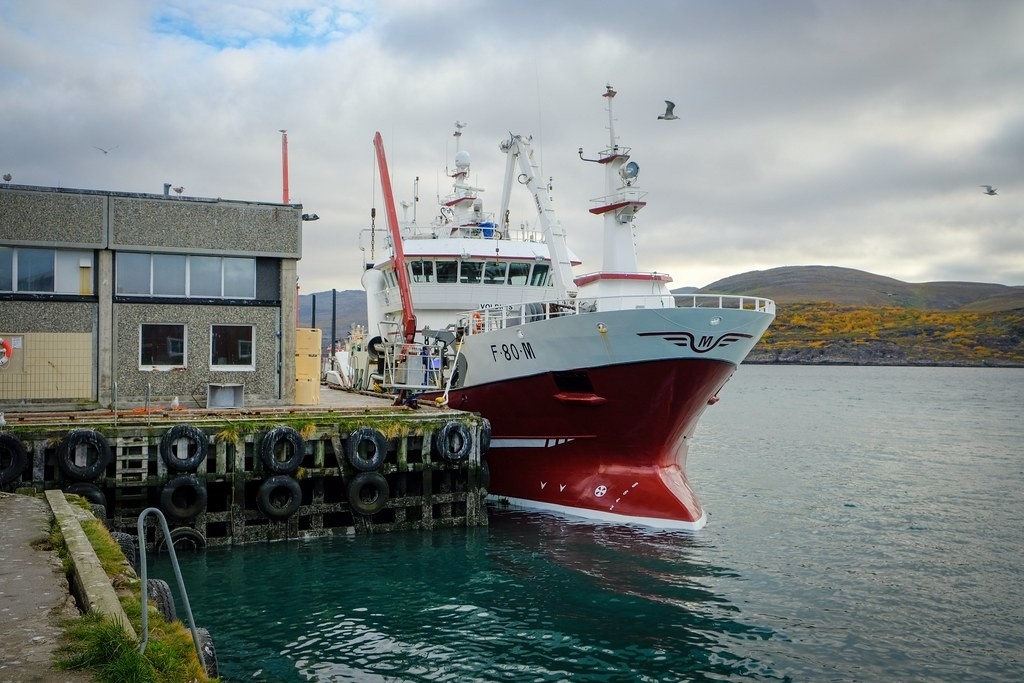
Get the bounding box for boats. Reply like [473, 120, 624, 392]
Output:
[320, 83, 776, 529]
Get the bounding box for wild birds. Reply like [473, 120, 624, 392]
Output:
[978, 185, 999, 195]
[3, 174, 12, 183]
[94, 145, 119, 153]
[173, 187, 185, 196]
[0, 412, 7, 431]
[171, 397, 179, 410]
[881, 292, 899, 296]
[658, 101, 680, 120]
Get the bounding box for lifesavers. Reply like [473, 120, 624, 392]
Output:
[259, 427, 307, 475]
[57, 428, 112, 482]
[160, 425, 209, 473]
[257, 476, 303, 518]
[0, 431, 32, 488]
[468, 311, 482, 333]
[156, 528, 208, 558]
[161, 476, 207, 522]
[346, 426, 389, 468]
[346, 472, 391, 517]
